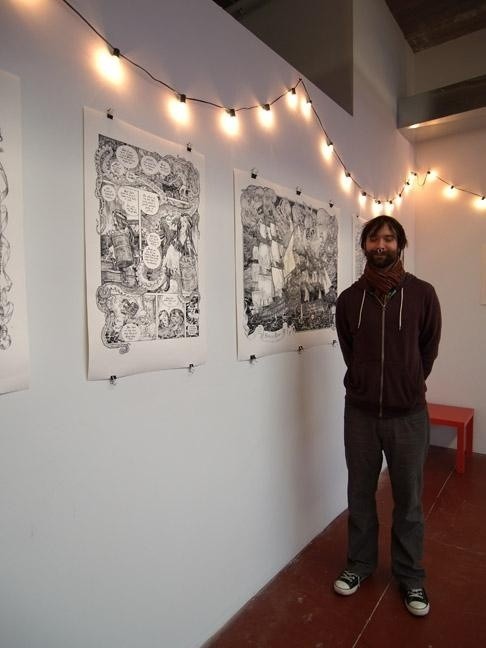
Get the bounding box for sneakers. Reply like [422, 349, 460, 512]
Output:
[334, 570, 367, 595]
[404, 585, 430, 616]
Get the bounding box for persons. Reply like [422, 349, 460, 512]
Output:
[332, 215, 443, 618]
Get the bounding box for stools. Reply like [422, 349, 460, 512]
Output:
[425, 400, 477, 475]
